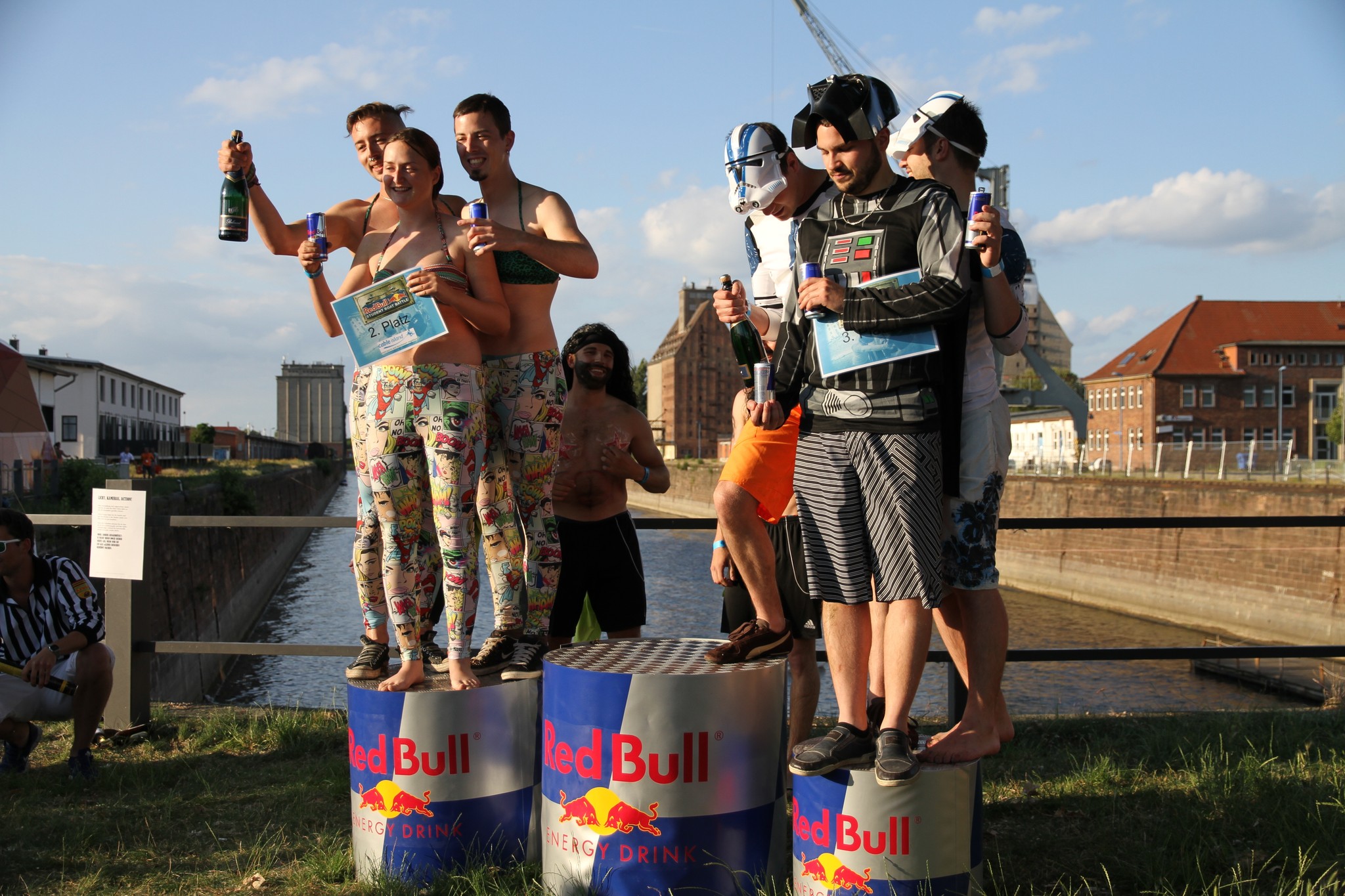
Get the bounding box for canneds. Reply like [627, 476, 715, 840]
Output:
[799, 261, 826, 319]
[754, 361, 775, 416]
[306, 212, 328, 261]
[469, 196, 489, 251]
[965, 187, 992, 250]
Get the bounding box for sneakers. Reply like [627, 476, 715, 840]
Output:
[471, 628, 524, 676]
[394, 630, 449, 673]
[501, 635, 549, 680]
[788, 722, 876, 776]
[867, 697, 919, 746]
[704, 619, 793, 665]
[874, 731, 920, 786]
[346, 634, 390, 680]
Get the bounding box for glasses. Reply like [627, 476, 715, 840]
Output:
[0, 539, 21, 553]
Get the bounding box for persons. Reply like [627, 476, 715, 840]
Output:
[219, 75, 1029, 786]
[54, 441, 158, 481]
[0, 509, 115, 784]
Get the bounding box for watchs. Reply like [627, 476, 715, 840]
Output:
[44, 643, 60, 659]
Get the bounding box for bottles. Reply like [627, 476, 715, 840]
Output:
[720, 275, 770, 387]
[218, 131, 249, 243]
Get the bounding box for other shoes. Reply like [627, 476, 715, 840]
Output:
[67, 746, 93, 781]
[0, 722, 43, 774]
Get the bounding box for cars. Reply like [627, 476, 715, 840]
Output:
[1089, 458, 1112, 475]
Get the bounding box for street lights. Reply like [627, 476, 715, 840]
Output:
[1278, 365, 1287, 475]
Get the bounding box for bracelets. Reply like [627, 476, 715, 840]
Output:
[745, 298, 751, 317]
[980, 257, 1005, 278]
[248, 176, 261, 189]
[634, 466, 649, 484]
[304, 261, 323, 279]
[244, 160, 256, 182]
[713, 539, 726, 549]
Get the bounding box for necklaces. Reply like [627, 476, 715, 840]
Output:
[841, 173, 896, 224]
[379, 191, 393, 201]
